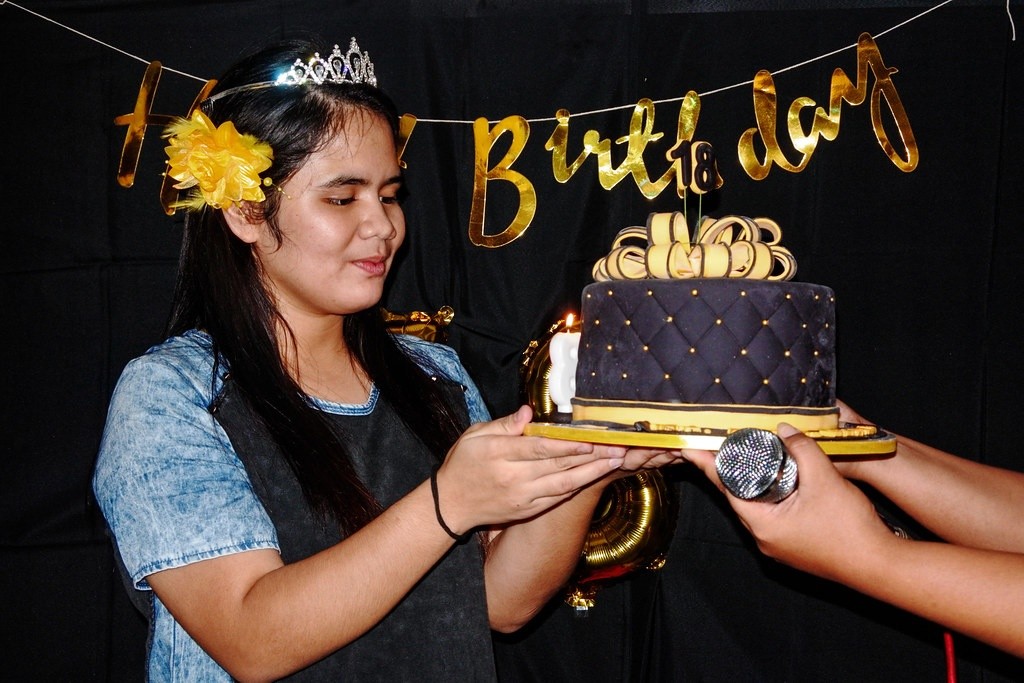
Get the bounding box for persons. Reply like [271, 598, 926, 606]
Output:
[88, 36, 687, 682]
[684, 397, 1023, 661]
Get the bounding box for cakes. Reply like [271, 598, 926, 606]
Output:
[569, 277, 840, 431]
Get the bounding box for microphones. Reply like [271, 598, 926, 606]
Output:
[716, 427, 913, 542]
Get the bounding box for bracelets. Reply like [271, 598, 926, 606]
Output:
[429, 464, 467, 541]
